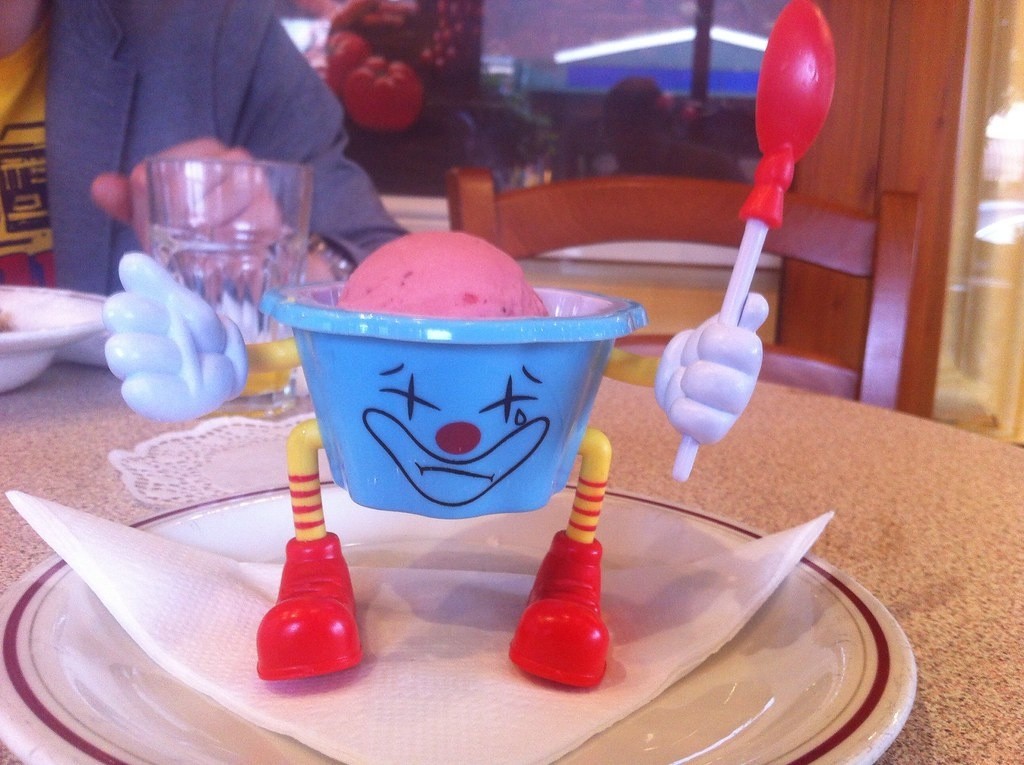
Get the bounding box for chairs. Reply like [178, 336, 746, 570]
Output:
[447, 162, 921, 409]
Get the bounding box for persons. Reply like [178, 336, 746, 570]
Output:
[0, 1, 414, 303]
[373, 70, 777, 202]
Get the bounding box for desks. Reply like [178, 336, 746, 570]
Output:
[0, 350, 1024, 765]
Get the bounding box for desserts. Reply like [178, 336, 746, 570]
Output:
[338, 231, 546, 321]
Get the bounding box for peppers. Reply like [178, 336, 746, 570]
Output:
[305, 31, 368, 96]
[345, 54, 421, 132]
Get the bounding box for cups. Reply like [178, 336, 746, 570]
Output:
[143, 151, 309, 424]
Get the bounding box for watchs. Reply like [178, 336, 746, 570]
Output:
[306, 232, 354, 282]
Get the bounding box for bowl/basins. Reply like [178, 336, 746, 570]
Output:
[262, 273, 647, 520]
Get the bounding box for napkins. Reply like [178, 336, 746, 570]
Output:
[3, 490, 838, 765]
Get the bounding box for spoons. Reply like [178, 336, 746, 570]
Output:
[674, 0, 837, 483]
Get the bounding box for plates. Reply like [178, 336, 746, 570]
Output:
[0, 284, 112, 395]
[0, 478, 923, 765]
